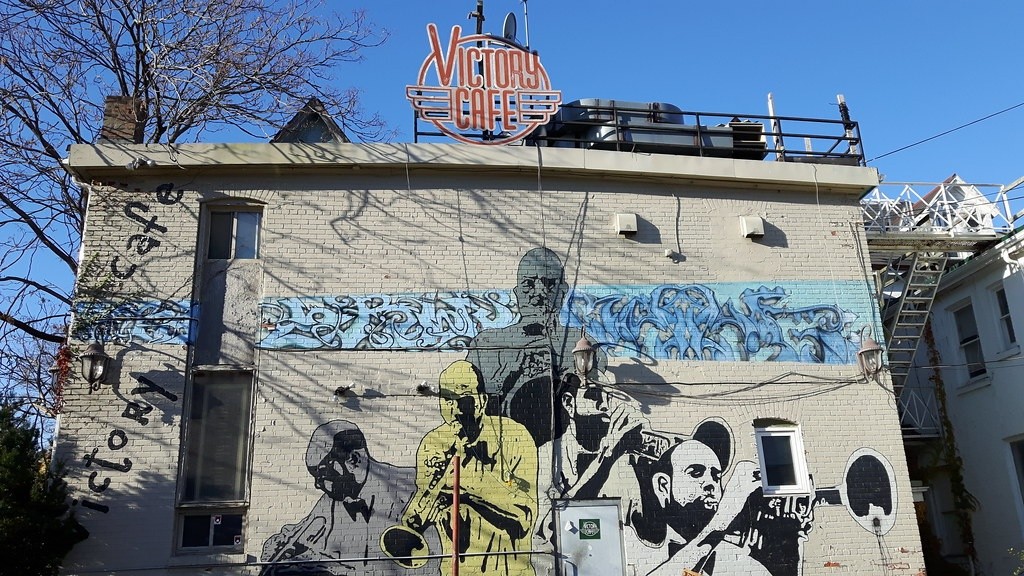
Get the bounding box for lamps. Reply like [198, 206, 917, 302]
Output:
[858, 325, 886, 382]
[572, 325, 595, 388]
[48, 356, 63, 396]
[79, 325, 112, 396]
